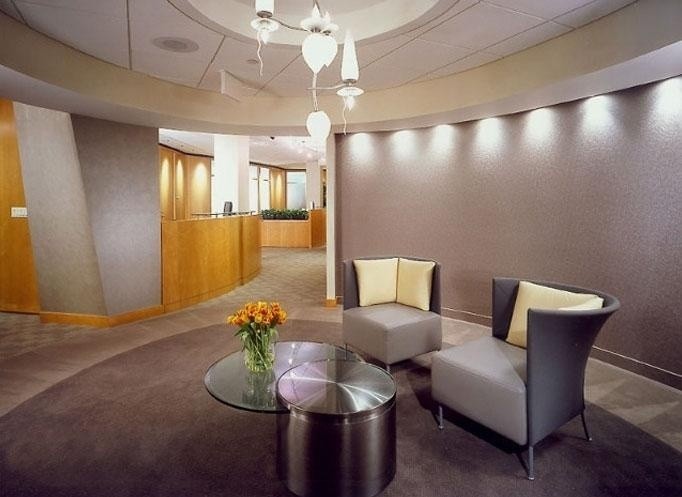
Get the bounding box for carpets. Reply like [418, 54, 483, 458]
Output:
[1, 316, 681, 497]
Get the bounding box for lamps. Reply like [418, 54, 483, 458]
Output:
[248, 0, 366, 163]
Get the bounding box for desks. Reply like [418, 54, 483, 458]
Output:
[203, 335, 399, 497]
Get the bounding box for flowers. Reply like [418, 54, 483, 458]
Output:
[224, 298, 289, 371]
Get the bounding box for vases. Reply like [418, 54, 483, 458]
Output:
[239, 329, 280, 370]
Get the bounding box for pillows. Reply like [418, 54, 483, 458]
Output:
[352, 257, 398, 306]
[559, 296, 605, 316]
[504, 279, 601, 348]
[397, 257, 438, 311]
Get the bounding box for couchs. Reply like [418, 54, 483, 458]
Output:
[341, 255, 443, 372]
[427, 275, 624, 479]
